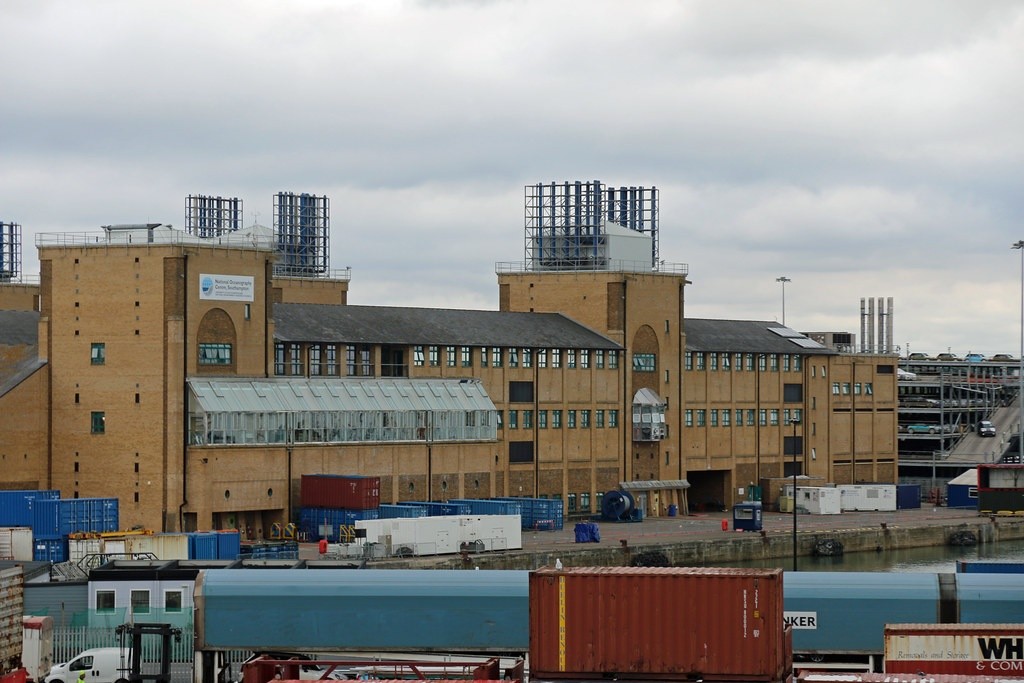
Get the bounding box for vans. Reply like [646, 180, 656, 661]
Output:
[45, 647, 142, 683]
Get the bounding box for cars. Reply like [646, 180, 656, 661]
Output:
[977, 421, 996, 437]
[937, 353, 962, 361]
[993, 354, 1021, 362]
[898, 368, 917, 381]
[898, 397, 990, 407]
[898, 423, 959, 434]
[909, 353, 934, 360]
[964, 354, 987, 362]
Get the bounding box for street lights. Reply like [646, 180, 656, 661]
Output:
[776, 277, 791, 326]
[907, 343, 909, 372]
[1011, 240, 1024, 464]
[789, 417, 799, 571]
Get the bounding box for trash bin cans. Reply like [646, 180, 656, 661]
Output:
[732, 501, 762, 532]
[668, 504, 676, 516]
[574, 523, 600, 543]
